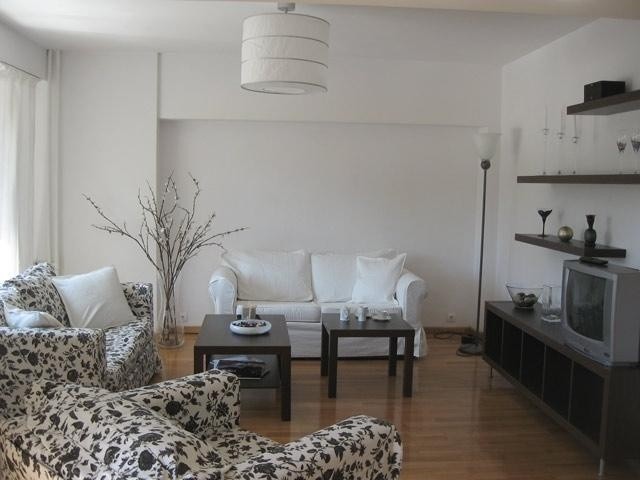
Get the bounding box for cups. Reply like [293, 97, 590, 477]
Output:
[339, 305, 352, 321]
[241, 304, 258, 320]
[375, 309, 389, 319]
[356, 306, 368, 322]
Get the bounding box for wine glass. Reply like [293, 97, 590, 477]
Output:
[615, 129, 640, 174]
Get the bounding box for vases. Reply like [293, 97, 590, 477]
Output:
[152, 267, 185, 350]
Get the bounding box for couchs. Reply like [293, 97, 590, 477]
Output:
[2, 371, 402, 480]
[0, 262, 162, 418]
[210, 248, 430, 359]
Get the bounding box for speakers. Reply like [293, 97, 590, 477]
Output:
[584, 81, 625, 103]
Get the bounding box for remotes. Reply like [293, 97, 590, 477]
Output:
[580, 256, 608, 265]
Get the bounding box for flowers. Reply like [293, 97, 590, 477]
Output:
[83, 167, 250, 276]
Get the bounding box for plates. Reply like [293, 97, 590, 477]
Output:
[374, 316, 392, 321]
[354, 313, 372, 318]
[236, 314, 260, 319]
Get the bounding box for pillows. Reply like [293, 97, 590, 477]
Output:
[48, 265, 137, 331]
[350, 253, 409, 306]
[1, 300, 61, 329]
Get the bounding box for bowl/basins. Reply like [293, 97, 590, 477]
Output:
[230, 319, 271, 335]
[504, 282, 543, 307]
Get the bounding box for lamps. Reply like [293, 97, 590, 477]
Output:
[237, 1, 331, 97]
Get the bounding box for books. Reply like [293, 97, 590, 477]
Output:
[207, 357, 271, 381]
[210, 356, 271, 376]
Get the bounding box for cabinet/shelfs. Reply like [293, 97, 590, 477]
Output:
[512, 83, 639, 261]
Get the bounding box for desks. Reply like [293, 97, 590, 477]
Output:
[484, 299, 639, 478]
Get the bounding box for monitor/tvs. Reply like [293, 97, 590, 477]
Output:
[561, 261, 640, 367]
